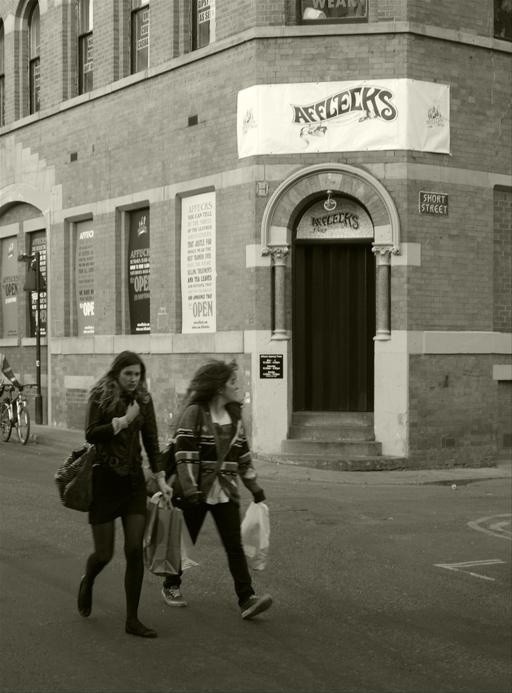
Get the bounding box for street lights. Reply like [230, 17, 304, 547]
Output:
[15, 250, 45, 423]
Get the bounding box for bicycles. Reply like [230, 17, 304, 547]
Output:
[0, 383, 41, 445]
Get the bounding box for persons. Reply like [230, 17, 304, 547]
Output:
[1, 353, 24, 416]
[161, 356, 272, 619]
[76, 349, 164, 639]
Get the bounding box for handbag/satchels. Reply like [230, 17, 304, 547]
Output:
[54, 442, 97, 514]
[237, 500, 272, 573]
[142, 492, 201, 576]
[144, 441, 203, 508]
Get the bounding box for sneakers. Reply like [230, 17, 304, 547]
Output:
[124, 619, 157, 639]
[77, 572, 96, 618]
[240, 592, 274, 620]
[161, 585, 188, 607]
